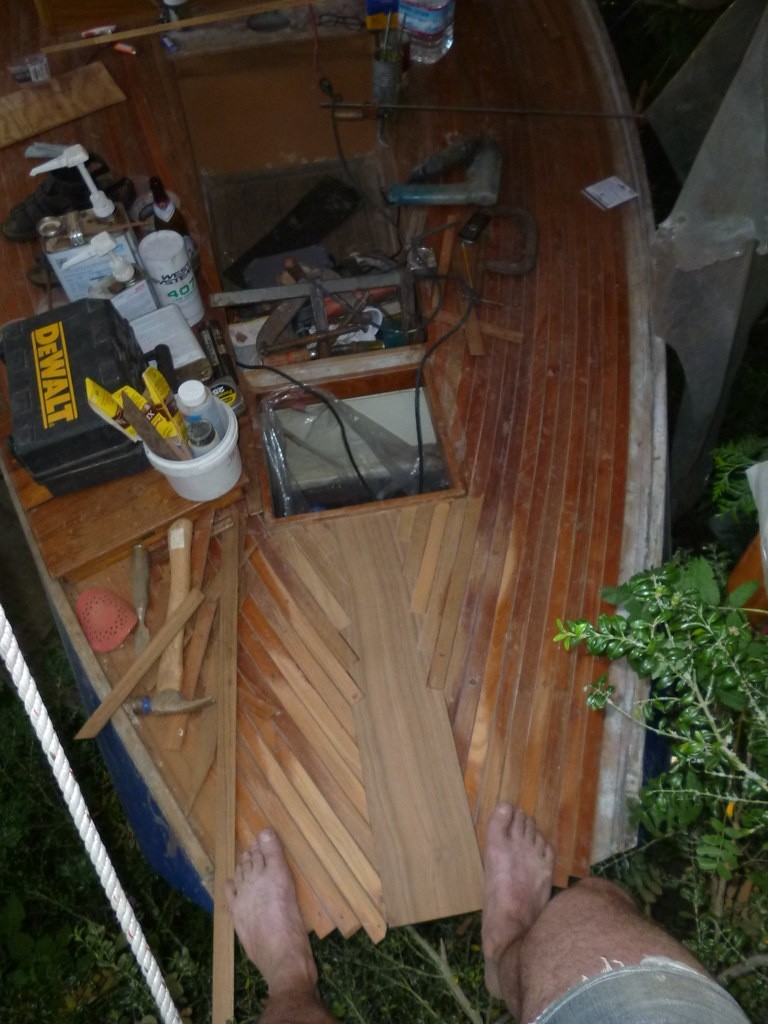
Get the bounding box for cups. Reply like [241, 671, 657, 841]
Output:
[130, 190, 180, 234]
[25, 53, 51, 85]
[6, 57, 31, 87]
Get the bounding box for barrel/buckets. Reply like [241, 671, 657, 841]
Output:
[143, 392, 242, 502]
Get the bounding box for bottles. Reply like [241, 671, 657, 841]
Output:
[398, 0, 455, 64]
[150, 175, 201, 274]
[176, 380, 226, 441]
[188, 421, 220, 457]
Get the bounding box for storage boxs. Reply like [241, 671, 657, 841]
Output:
[0, 298, 149, 497]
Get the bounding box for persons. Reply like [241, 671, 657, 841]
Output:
[226, 803, 750, 1024]
